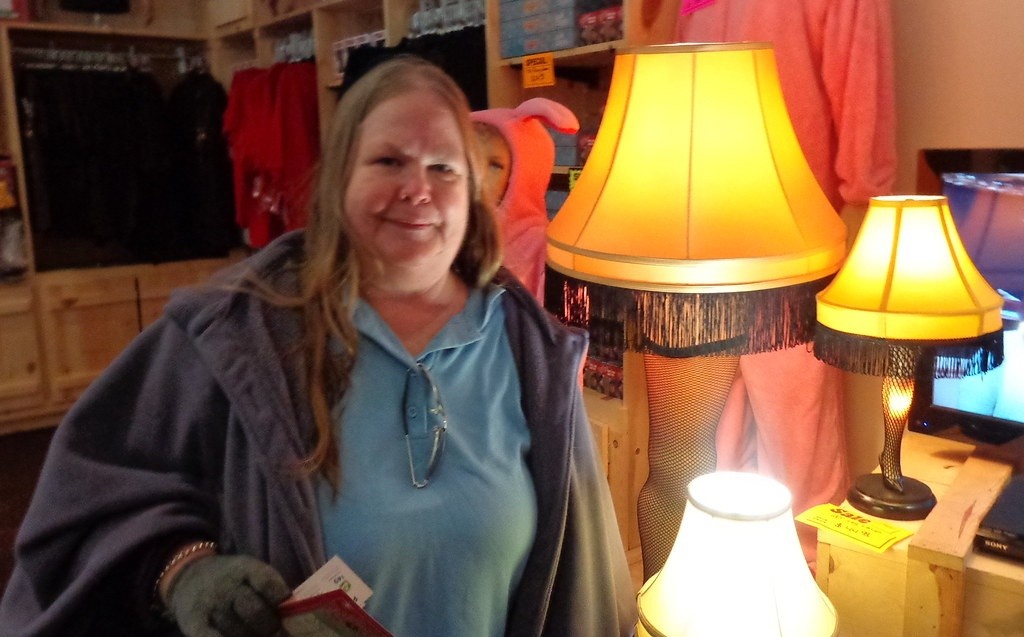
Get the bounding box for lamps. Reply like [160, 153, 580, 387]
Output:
[540, 39, 847, 598]
[809, 192, 1004, 521]
[632, 469, 841, 637]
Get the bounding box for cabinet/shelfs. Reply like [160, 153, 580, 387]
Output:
[0, 0, 679, 557]
[814, 429, 1023, 637]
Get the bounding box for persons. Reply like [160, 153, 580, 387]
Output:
[0, 59, 638, 637]
[469, 98, 581, 305]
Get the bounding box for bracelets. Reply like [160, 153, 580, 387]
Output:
[153, 538, 216, 593]
